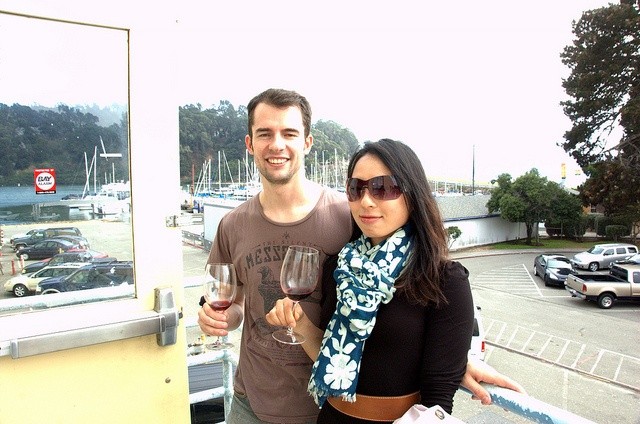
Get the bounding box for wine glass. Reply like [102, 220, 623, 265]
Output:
[271, 245, 320, 345]
[203, 263, 238, 350]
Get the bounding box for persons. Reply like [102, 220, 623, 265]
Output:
[265, 139, 474, 424]
[197, 89, 526, 424]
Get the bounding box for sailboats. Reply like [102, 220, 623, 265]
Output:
[429, 180, 484, 198]
[182, 151, 266, 212]
[34, 135, 130, 223]
[306, 149, 350, 193]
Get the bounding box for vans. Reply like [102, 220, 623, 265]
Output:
[569, 243, 638, 273]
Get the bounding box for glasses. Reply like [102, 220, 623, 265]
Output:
[345, 174, 401, 201]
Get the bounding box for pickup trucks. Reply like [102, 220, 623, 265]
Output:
[562, 264, 640, 307]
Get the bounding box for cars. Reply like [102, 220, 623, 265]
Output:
[607, 251, 640, 273]
[534, 254, 573, 289]
[5, 224, 134, 298]
